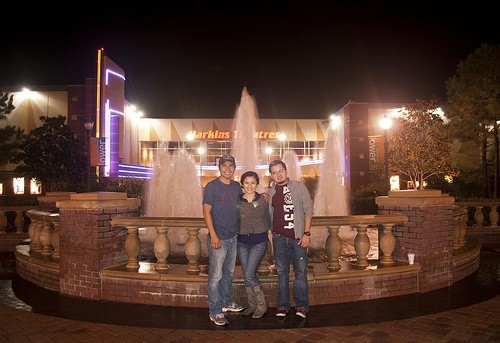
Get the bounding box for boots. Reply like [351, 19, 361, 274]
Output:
[252, 284, 267, 319]
[242, 287, 258, 316]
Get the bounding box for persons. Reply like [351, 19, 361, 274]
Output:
[237, 171, 274, 318]
[267, 160, 314, 319]
[202, 154, 270, 325]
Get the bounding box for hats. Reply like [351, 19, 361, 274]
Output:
[219, 154, 234, 164]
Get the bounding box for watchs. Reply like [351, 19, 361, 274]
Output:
[304, 231, 311, 236]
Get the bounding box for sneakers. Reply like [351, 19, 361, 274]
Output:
[210, 312, 229, 326]
[221, 302, 244, 312]
[296, 307, 306, 318]
[275, 307, 287, 317]
